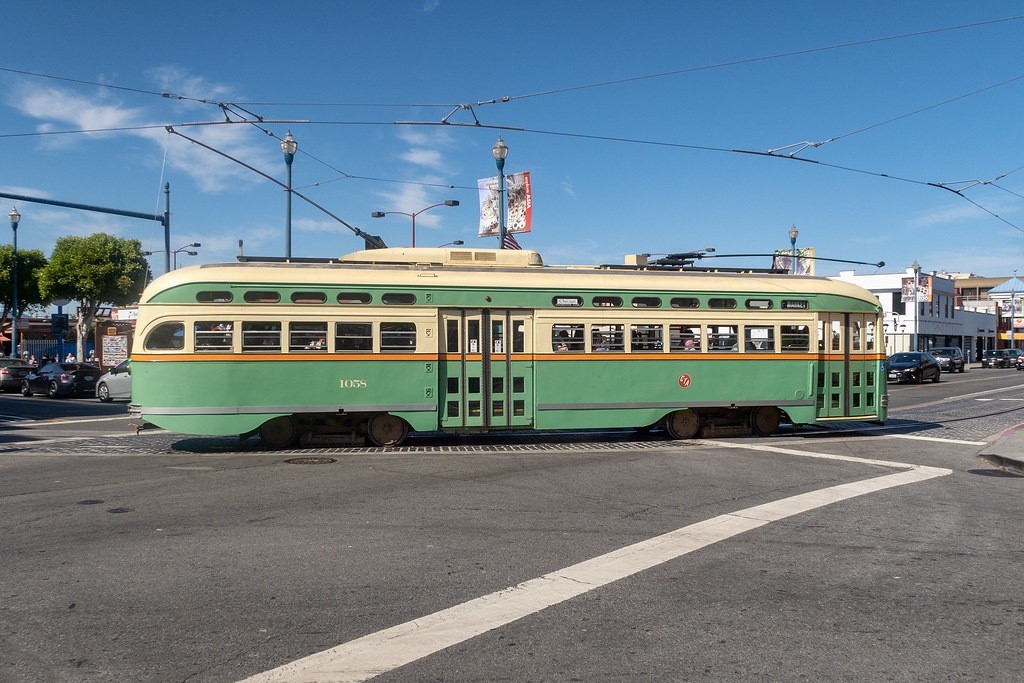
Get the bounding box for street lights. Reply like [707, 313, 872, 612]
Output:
[1011, 289, 1015, 350]
[371, 200, 460, 248]
[142, 242, 201, 271]
[8, 205, 21, 358]
[491, 132, 508, 249]
[912, 259, 919, 351]
[280, 129, 298, 258]
[789, 223, 799, 275]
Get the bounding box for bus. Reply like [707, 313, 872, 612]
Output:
[127, 125, 890, 447]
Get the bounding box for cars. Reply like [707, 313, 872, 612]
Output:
[1006, 348, 1024, 371]
[95, 359, 131, 403]
[0, 358, 37, 393]
[20, 362, 102, 400]
[982, 350, 1010, 369]
[886, 352, 941, 385]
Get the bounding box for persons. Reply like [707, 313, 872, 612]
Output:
[555, 326, 809, 351]
[28, 353, 75, 367]
[198, 323, 414, 350]
[0, 344, 5, 358]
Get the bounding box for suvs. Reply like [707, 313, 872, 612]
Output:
[928, 347, 965, 373]
